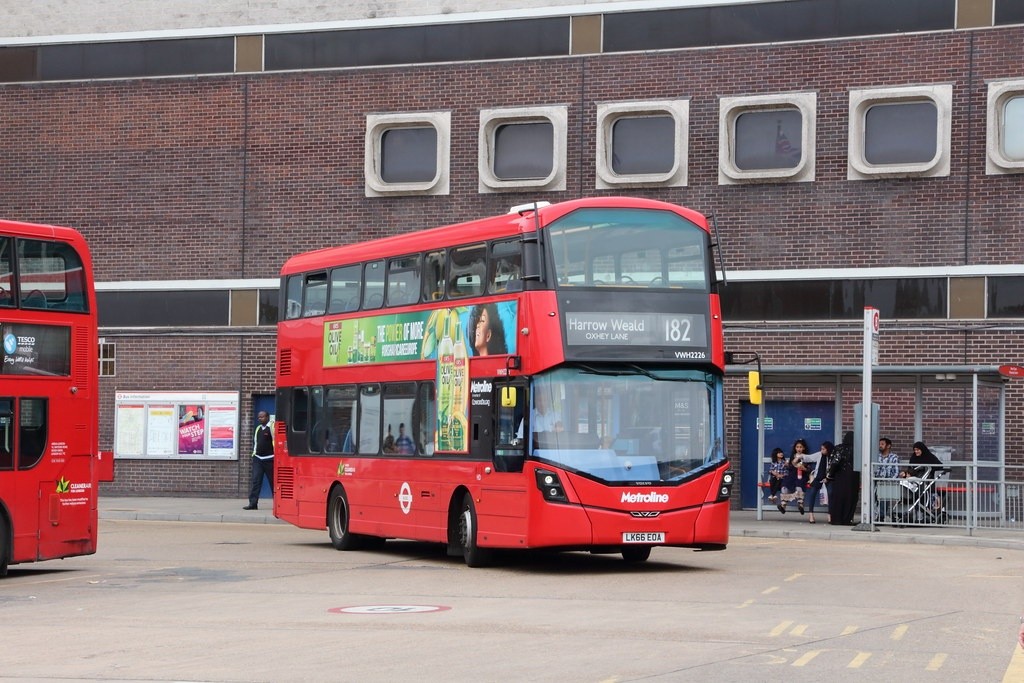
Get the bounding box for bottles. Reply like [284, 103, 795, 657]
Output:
[792, 462, 806, 470]
[449, 322, 469, 453]
[435, 317, 454, 454]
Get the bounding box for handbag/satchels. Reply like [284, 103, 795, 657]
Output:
[832, 458, 851, 491]
[819, 482, 829, 506]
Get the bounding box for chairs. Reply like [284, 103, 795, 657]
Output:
[0, 286, 87, 312]
[308, 292, 418, 314]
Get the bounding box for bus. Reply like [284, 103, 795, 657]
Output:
[0, 220, 100, 576]
[273, 197, 765, 568]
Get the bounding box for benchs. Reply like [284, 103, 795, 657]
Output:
[760, 481, 810, 491]
[934, 486, 997, 495]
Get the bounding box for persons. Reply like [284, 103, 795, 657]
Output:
[822, 431, 860, 525]
[777, 439, 812, 515]
[875, 438, 900, 528]
[768, 448, 788, 504]
[899, 441, 943, 527]
[383, 423, 414, 454]
[796, 441, 834, 524]
[242, 412, 275, 509]
[469, 302, 508, 356]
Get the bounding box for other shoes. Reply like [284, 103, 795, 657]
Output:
[242, 503, 258, 510]
[828, 515, 832, 525]
[809, 516, 815, 523]
[799, 505, 804, 515]
[777, 504, 786, 514]
[768, 495, 777, 504]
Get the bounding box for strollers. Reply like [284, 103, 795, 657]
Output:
[891, 466, 951, 526]
